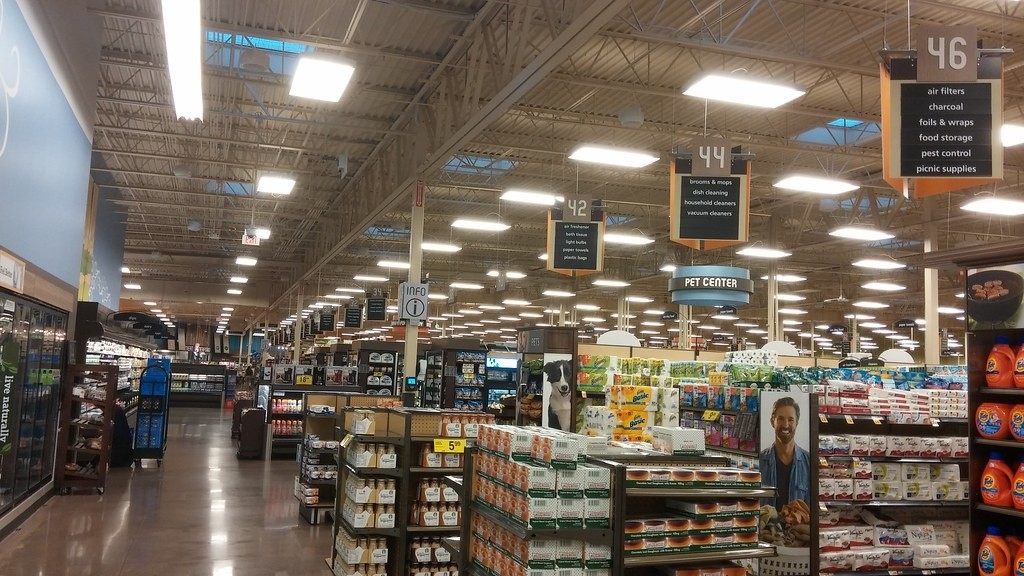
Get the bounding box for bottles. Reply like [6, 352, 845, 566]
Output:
[272, 420, 302, 435]
[412, 536, 441, 548]
[345, 497, 395, 514]
[443, 413, 495, 423]
[353, 410, 375, 421]
[338, 526, 386, 549]
[410, 561, 458, 573]
[981, 452, 1014, 508]
[411, 500, 461, 512]
[420, 443, 431, 454]
[986, 336, 1024, 389]
[1012, 455, 1024, 512]
[347, 471, 395, 490]
[978, 527, 1024, 576]
[272, 399, 302, 414]
[336, 553, 386, 574]
[419, 478, 448, 488]
[130, 396, 138, 405]
[350, 442, 395, 454]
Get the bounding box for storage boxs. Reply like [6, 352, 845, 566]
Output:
[652, 425, 705, 455]
[472, 426, 609, 576]
[135, 358, 172, 449]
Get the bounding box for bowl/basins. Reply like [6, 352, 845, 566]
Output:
[968, 270, 1024, 324]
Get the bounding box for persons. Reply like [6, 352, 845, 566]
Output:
[759, 397, 810, 513]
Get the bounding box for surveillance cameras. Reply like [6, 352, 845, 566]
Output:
[246, 229, 256, 238]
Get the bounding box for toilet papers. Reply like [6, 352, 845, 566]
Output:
[576, 354, 775, 393]
[583, 385, 680, 442]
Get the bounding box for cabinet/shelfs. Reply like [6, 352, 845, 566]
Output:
[587, 454, 778, 576]
[168, 363, 227, 409]
[299, 392, 414, 526]
[54, 365, 119, 494]
[949, 238, 1024, 576]
[77, 352, 148, 445]
[760, 391, 969, 576]
[258, 383, 361, 461]
[681, 406, 759, 457]
[325, 406, 478, 576]
[423, 339, 517, 424]
[351, 341, 430, 407]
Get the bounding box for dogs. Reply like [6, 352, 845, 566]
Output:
[544, 359, 573, 432]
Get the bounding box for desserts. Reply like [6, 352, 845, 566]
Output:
[624, 471, 760, 549]
